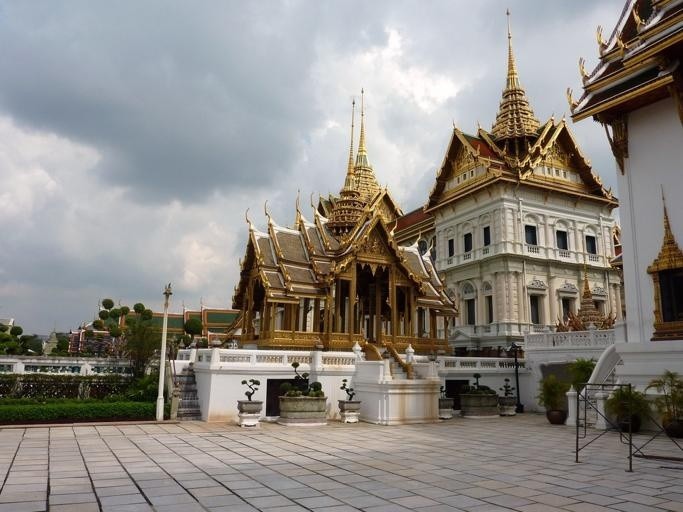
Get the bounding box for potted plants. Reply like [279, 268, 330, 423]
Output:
[459, 374, 498, 413]
[536, 375, 572, 424]
[338, 379, 362, 413]
[605, 382, 651, 433]
[237, 379, 264, 413]
[646, 369, 683, 438]
[279, 362, 329, 418]
[499, 378, 518, 406]
[439, 386, 455, 408]
